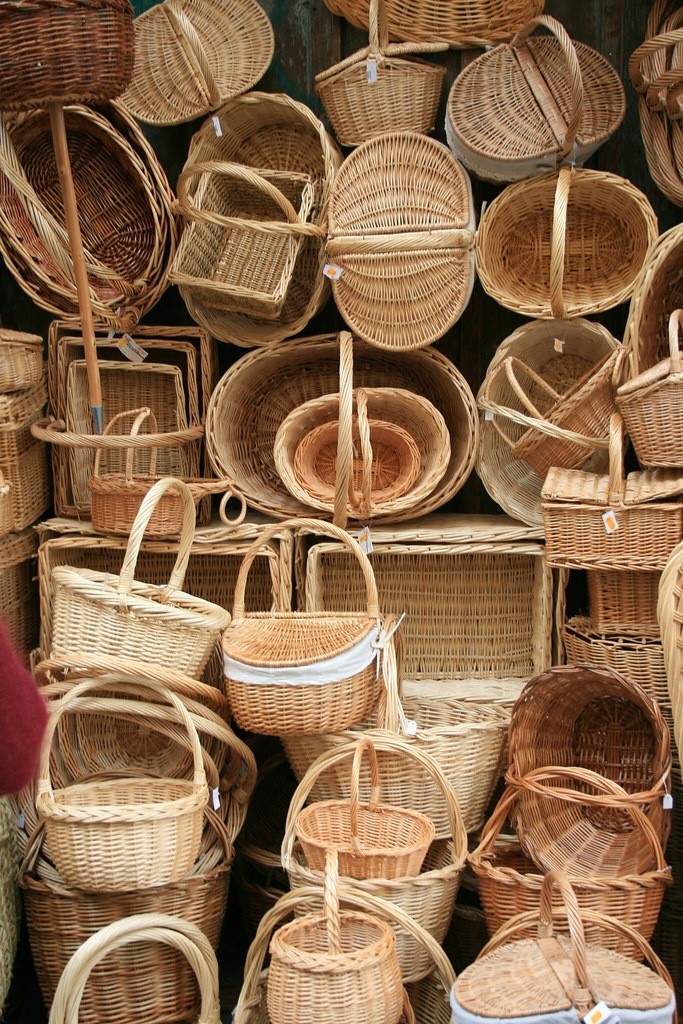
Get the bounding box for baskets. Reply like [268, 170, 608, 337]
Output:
[0, 0, 683, 1024]
